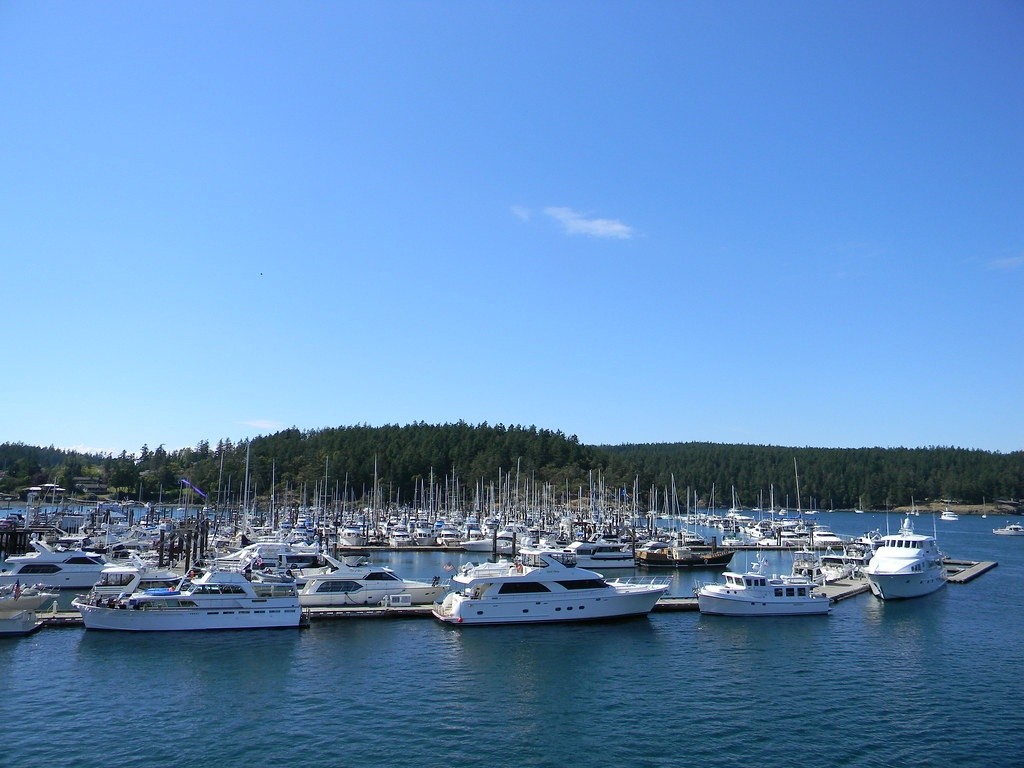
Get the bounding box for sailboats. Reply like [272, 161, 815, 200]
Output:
[981, 496, 988, 519]
[906, 494, 921, 516]
[1, 435, 876, 631]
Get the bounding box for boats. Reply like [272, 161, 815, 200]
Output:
[694, 553, 833, 616]
[992, 521, 1024, 536]
[433, 547, 674, 625]
[861, 517, 951, 600]
[68, 561, 311, 629]
[0, 583, 60, 635]
[940, 507, 959, 521]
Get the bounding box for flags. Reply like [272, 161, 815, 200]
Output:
[13, 578, 21, 601]
[443, 560, 455, 572]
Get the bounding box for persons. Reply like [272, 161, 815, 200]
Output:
[169, 585, 175, 592]
[255, 554, 263, 570]
[514, 562, 523, 573]
